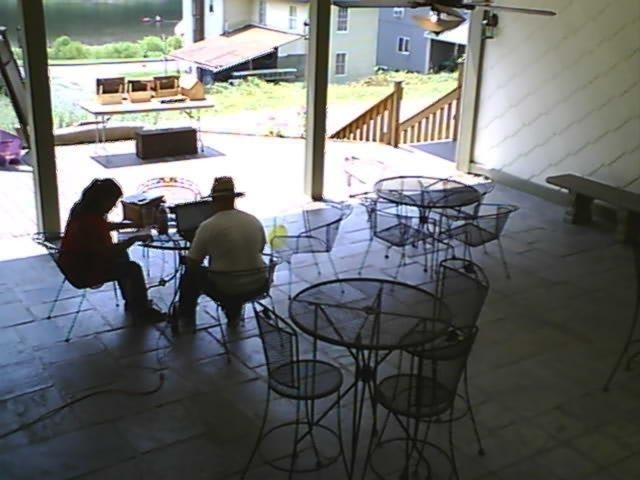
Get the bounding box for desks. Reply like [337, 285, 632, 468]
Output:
[374, 175, 482, 261]
[79, 98, 215, 152]
[137, 231, 192, 324]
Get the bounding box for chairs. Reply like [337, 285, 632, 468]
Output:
[240, 299, 350, 480]
[435, 203, 520, 281]
[602, 243, 639, 391]
[372, 259, 487, 458]
[268, 205, 354, 300]
[361, 324, 480, 480]
[132, 176, 203, 277]
[358, 201, 436, 291]
[428, 171, 495, 255]
[160, 253, 284, 365]
[31, 229, 147, 343]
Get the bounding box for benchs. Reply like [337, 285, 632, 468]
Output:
[546, 174, 640, 242]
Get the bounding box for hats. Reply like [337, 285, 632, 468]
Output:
[200, 177, 244, 200]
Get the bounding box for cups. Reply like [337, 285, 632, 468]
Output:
[141, 206, 156, 231]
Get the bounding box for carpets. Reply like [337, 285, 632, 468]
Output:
[93, 147, 225, 169]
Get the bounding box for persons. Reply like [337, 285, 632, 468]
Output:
[56, 178, 168, 323]
[176, 177, 269, 328]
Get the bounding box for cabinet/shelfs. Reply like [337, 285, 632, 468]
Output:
[134, 127, 198, 160]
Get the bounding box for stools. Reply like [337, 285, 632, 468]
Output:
[97, 77, 126, 105]
[154, 75, 180, 98]
[128, 80, 152, 103]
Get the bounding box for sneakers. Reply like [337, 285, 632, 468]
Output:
[122, 299, 153, 311]
[226, 317, 241, 327]
[134, 307, 167, 320]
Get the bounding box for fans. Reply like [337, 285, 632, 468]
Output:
[330, 0, 557, 37]
